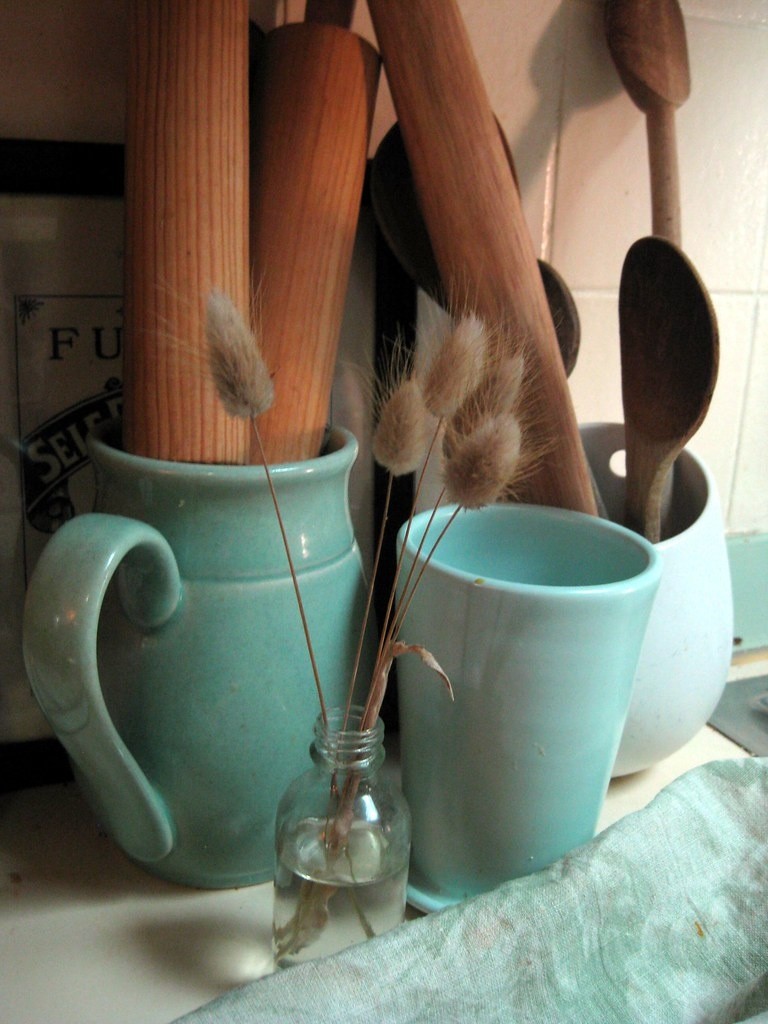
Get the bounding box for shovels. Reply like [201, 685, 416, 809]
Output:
[602, 1, 700, 255]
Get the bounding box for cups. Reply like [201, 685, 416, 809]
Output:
[580, 424, 729, 778]
[22, 415, 370, 889]
[394, 502, 661, 892]
